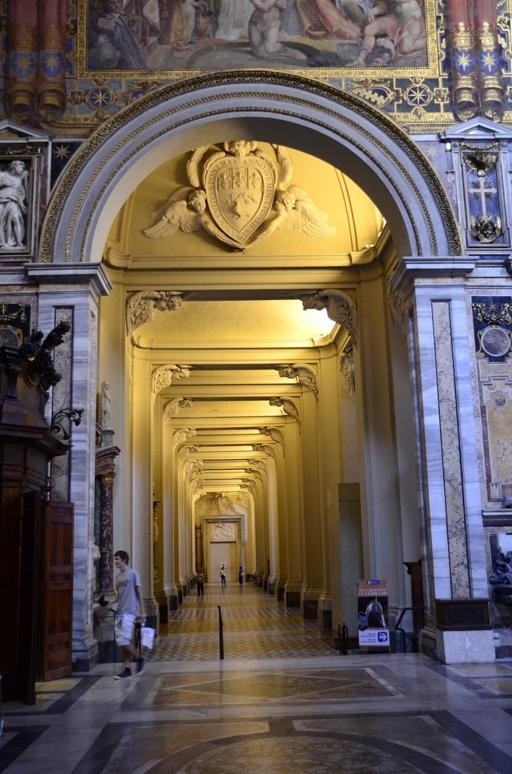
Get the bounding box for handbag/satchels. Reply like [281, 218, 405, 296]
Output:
[141, 627, 155, 649]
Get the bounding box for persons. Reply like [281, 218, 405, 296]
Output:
[239, 566, 243, 584]
[219, 566, 227, 587]
[106, 551, 143, 680]
[194, 570, 204, 597]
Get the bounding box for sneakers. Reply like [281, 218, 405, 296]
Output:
[137, 657, 145, 675]
[114, 671, 132, 679]
